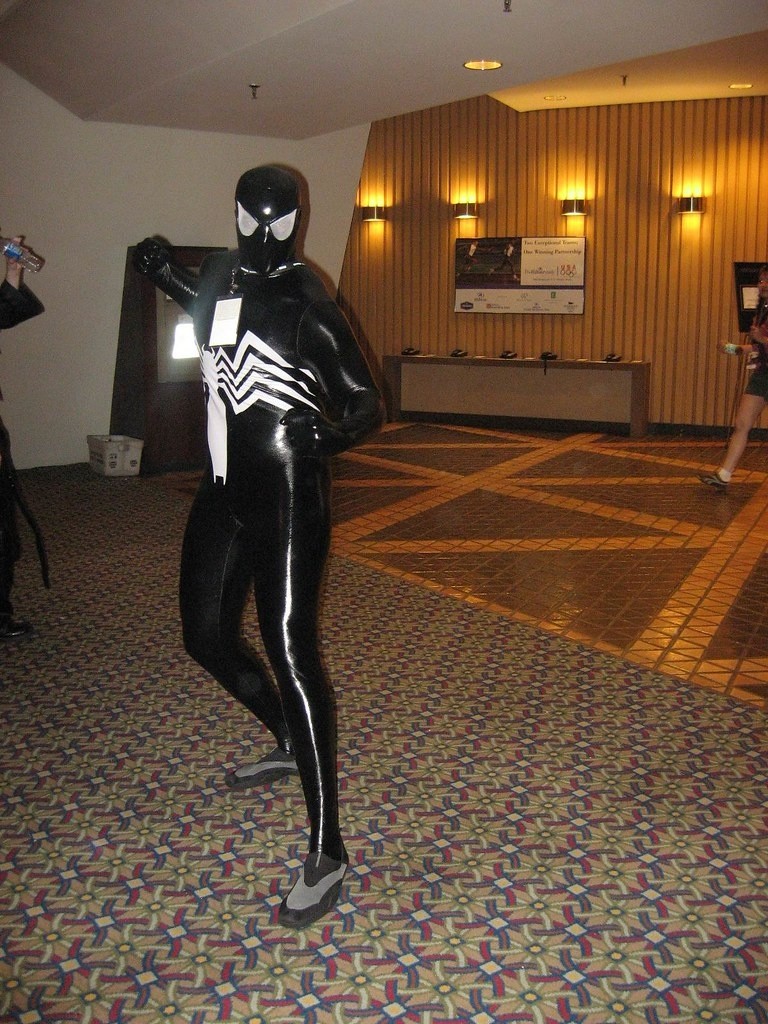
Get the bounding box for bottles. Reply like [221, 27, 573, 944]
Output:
[0, 236, 44, 273]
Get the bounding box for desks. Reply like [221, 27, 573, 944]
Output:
[384, 352, 651, 442]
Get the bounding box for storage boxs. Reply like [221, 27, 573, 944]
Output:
[86, 435, 143, 476]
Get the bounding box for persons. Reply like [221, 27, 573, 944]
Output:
[0, 228, 51, 638]
[489, 239, 520, 280]
[131, 165, 382, 929]
[456, 240, 478, 279]
[694, 266, 768, 490]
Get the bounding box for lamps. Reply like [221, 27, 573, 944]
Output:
[362, 206, 386, 222]
[563, 199, 589, 215]
[678, 197, 706, 214]
[452, 203, 478, 218]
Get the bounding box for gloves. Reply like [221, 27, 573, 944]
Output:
[132, 237, 168, 280]
[279, 408, 337, 457]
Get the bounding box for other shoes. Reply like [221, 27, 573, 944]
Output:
[277, 831, 348, 927]
[223, 747, 300, 788]
[695, 471, 729, 491]
